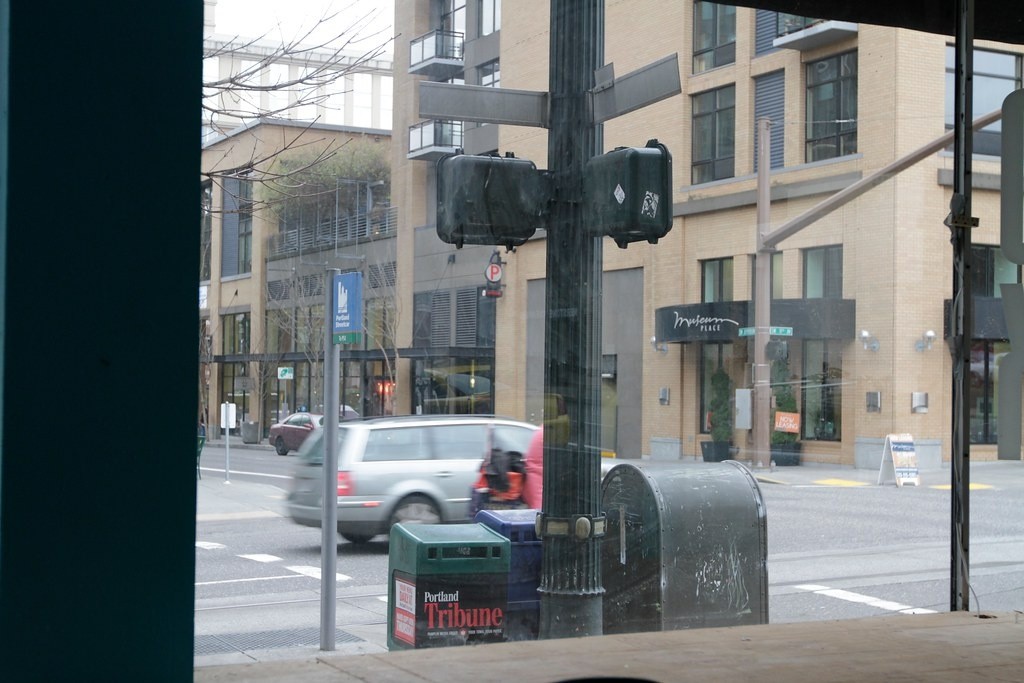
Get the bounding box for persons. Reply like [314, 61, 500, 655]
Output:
[521, 424, 543, 509]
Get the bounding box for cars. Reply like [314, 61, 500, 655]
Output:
[421, 374, 571, 449]
[267, 411, 362, 456]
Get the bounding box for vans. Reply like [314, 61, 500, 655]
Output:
[284, 414, 615, 544]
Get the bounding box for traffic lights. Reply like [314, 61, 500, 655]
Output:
[767, 339, 787, 362]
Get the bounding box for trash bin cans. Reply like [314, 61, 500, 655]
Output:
[241, 422, 264, 444]
[476, 508, 544, 642]
[386, 522, 510, 650]
[599, 459, 770, 636]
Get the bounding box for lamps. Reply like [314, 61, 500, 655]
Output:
[861, 328, 880, 352]
[915, 330, 937, 352]
